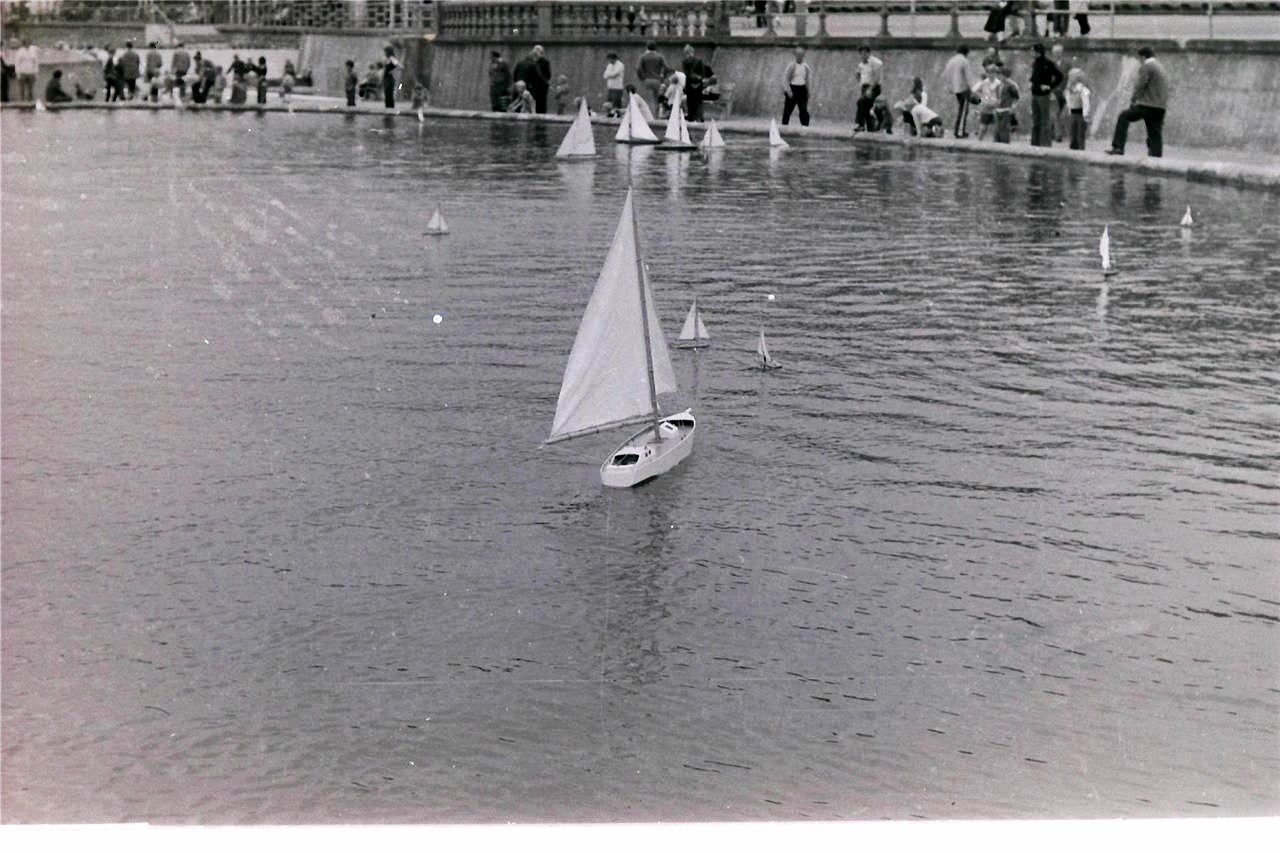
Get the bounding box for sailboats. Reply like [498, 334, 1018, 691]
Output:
[767, 115, 791, 149]
[553, 99, 605, 161]
[542, 185, 699, 490]
[676, 307, 711, 351]
[1096, 224, 1120, 278]
[751, 331, 782, 370]
[652, 88, 696, 149]
[614, 92, 660, 143]
[698, 115, 728, 150]
[421, 202, 452, 237]
[1179, 205, 1197, 227]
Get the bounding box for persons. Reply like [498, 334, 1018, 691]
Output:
[852, 47, 883, 130]
[893, 100, 917, 137]
[912, 77, 927, 106]
[1029, 43, 1064, 145]
[943, 43, 974, 138]
[744, 0, 810, 28]
[780, 45, 810, 128]
[1051, 44, 1072, 142]
[905, 98, 943, 138]
[0, 33, 721, 124]
[1064, 68, 1091, 151]
[973, 46, 1021, 144]
[872, 94, 892, 134]
[1104, 46, 1170, 158]
[984, 0, 1091, 40]
